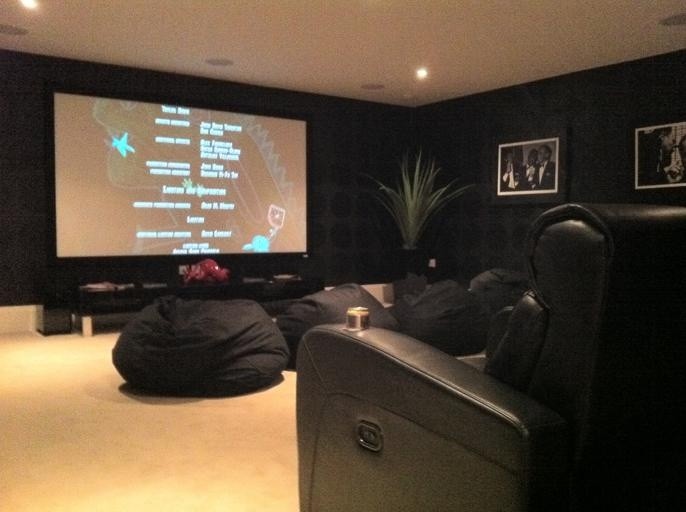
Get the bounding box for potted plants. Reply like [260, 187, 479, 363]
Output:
[361, 145, 475, 276]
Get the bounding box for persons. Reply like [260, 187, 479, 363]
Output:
[500, 148, 523, 191]
[531, 144, 556, 190]
[522, 147, 539, 190]
[638, 127, 686, 186]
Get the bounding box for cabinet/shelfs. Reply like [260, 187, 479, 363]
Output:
[71, 271, 306, 337]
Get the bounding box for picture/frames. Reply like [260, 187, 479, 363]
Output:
[623, 113, 686, 197]
[489, 125, 571, 207]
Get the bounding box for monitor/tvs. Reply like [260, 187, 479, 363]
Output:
[43, 81, 315, 269]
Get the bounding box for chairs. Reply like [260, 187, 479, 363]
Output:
[297, 202, 686, 511]
